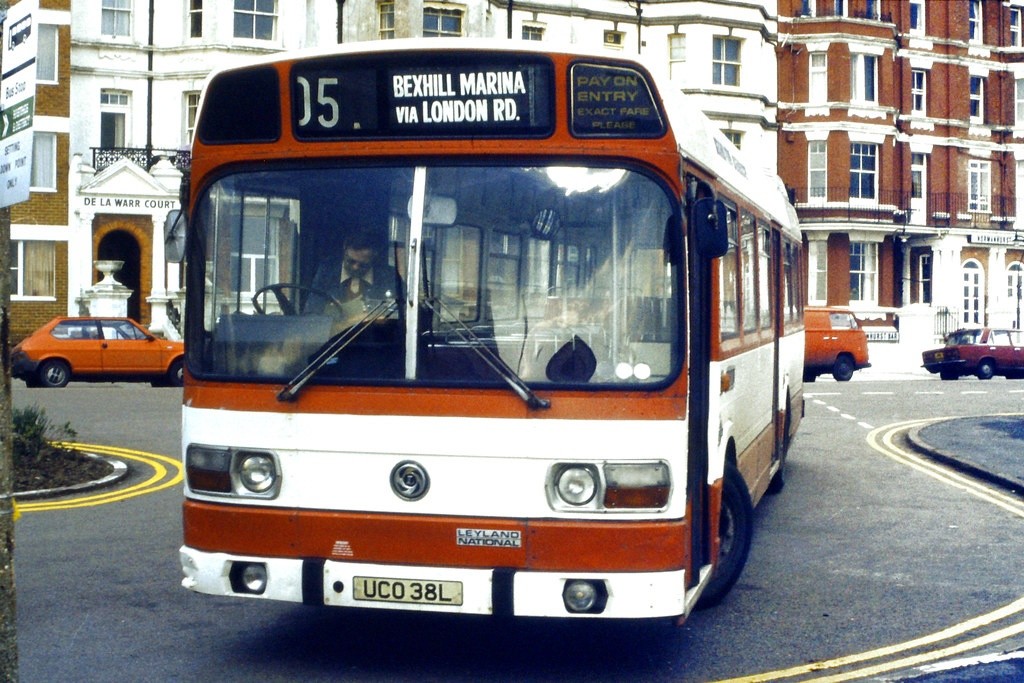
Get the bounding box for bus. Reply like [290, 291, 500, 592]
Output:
[163, 35, 804, 635]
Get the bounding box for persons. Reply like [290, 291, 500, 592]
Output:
[303, 232, 403, 341]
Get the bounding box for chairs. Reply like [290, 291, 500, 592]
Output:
[437, 291, 739, 343]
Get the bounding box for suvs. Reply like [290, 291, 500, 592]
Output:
[804, 306, 870, 382]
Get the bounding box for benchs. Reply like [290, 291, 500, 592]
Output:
[535, 286, 633, 356]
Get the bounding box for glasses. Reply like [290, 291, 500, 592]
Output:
[345, 252, 375, 269]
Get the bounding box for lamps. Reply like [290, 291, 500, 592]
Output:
[899, 234, 911, 243]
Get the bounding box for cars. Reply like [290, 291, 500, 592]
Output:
[921, 328, 1024, 379]
[11, 315, 185, 388]
